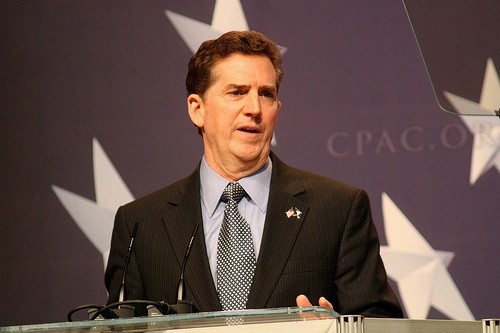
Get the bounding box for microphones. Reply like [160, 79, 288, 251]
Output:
[87, 221, 139, 320]
[165, 222, 200, 315]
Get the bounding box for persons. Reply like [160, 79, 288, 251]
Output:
[103, 29, 405, 319]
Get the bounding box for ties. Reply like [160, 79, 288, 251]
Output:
[216, 183, 256, 326]
